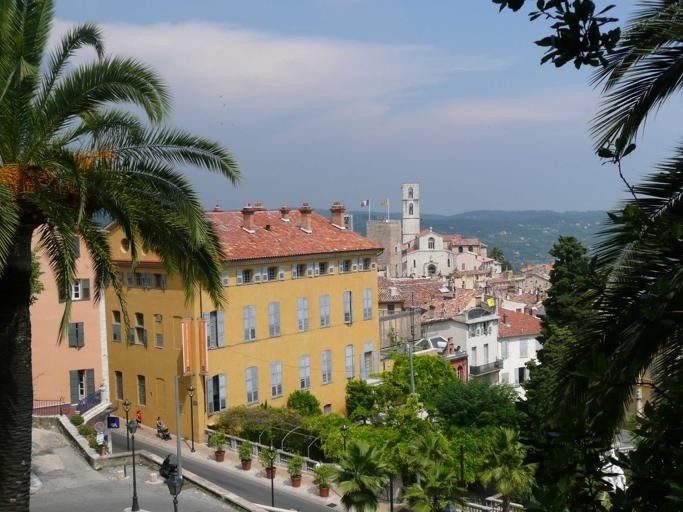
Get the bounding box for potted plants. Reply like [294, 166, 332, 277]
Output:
[286, 452, 303, 488]
[312, 461, 338, 498]
[259, 447, 278, 480]
[209, 432, 225, 463]
[238, 441, 253, 472]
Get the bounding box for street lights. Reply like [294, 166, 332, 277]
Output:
[408, 345, 423, 392]
[166, 465, 182, 511]
[187, 386, 199, 455]
[122, 399, 131, 454]
[172, 374, 184, 483]
[267, 445, 277, 508]
[123, 421, 147, 512]
[340, 424, 347, 452]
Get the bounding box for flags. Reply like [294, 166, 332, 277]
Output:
[487, 298, 495, 308]
[380, 199, 390, 208]
[360, 200, 370, 209]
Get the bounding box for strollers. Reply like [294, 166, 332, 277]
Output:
[156, 427, 171, 441]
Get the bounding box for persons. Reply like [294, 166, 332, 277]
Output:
[156, 416, 163, 437]
[135, 409, 143, 430]
[165, 430, 171, 437]
